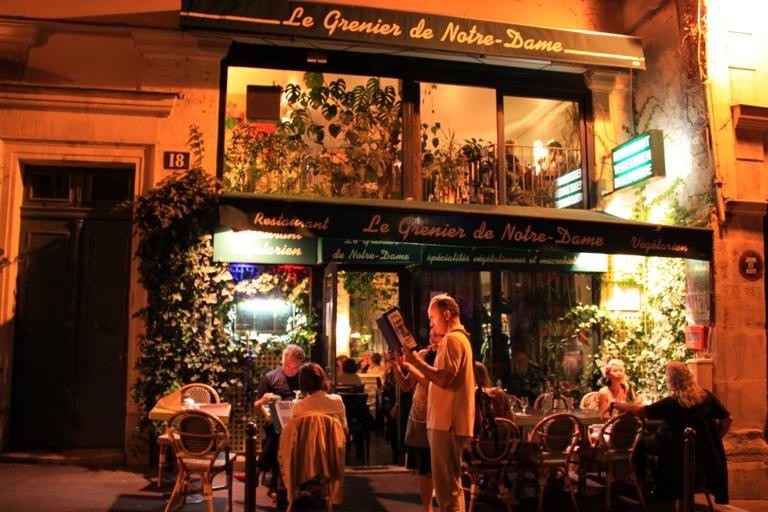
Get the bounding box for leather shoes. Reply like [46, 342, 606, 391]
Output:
[269, 491, 277, 499]
[234, 471, 246, 481]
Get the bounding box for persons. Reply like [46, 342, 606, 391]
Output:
[233, 362, 350, 501]
[591, 360, 642, 446]
[252, 343, 305, 411]
[602, 362, 734, 512]
[337, 294, 476, 512]
[506, 139, 577, 189]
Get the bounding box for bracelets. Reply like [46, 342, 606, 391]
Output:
[611, 400, 616, 409]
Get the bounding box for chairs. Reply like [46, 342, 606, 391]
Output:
[456, 383, 720, 512]
[145, 361, 397, 512]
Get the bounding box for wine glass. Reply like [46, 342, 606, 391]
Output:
[506, 394, 529, 414]
[292, 390, 301, 402]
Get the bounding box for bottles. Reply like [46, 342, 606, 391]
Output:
[542, 380, 561, 413]
